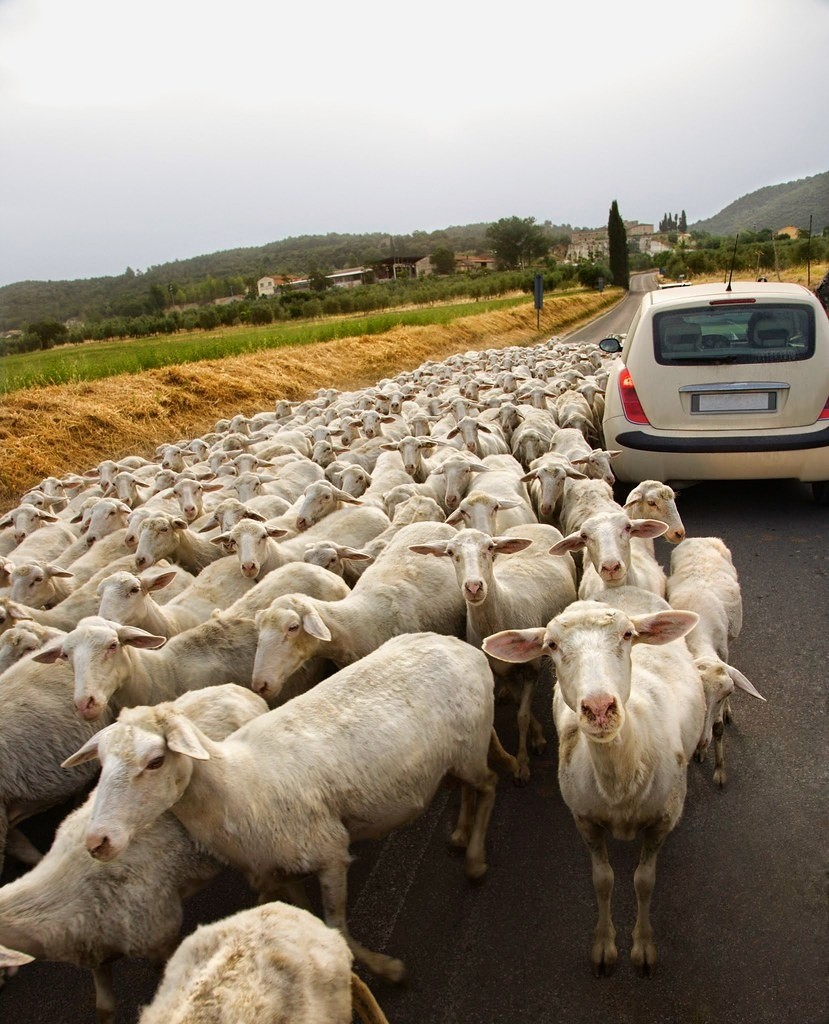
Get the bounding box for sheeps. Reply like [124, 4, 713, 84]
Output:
[1, 335, 767, 789]
[111, 901, 393, 1024]
[59, 631, 504, 978]
[1, 799, 321, 1024]
[479, 586, 707, 981]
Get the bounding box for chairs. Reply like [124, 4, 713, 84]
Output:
[667, 323, 706, 355]
[753, 319, 797, 348]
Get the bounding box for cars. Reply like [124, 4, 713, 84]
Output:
[599, 281, 829, 511]
